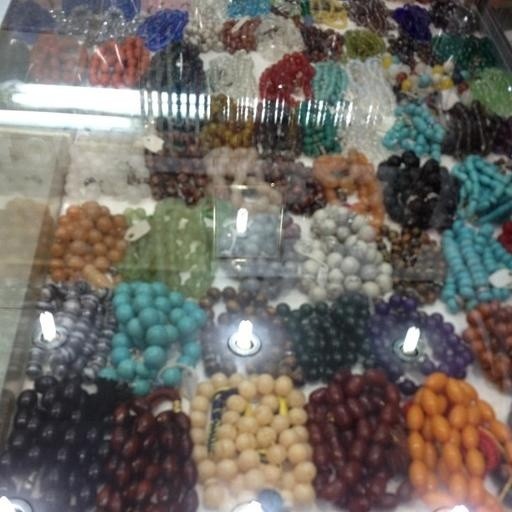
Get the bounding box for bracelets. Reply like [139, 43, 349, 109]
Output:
[1, 0, 511, 512]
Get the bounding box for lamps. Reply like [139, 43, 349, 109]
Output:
[228, 320, 261, 356]
[230, 207, 252, 238]
[32, 310, 69, 351]
[393, 326, 421, 362]
